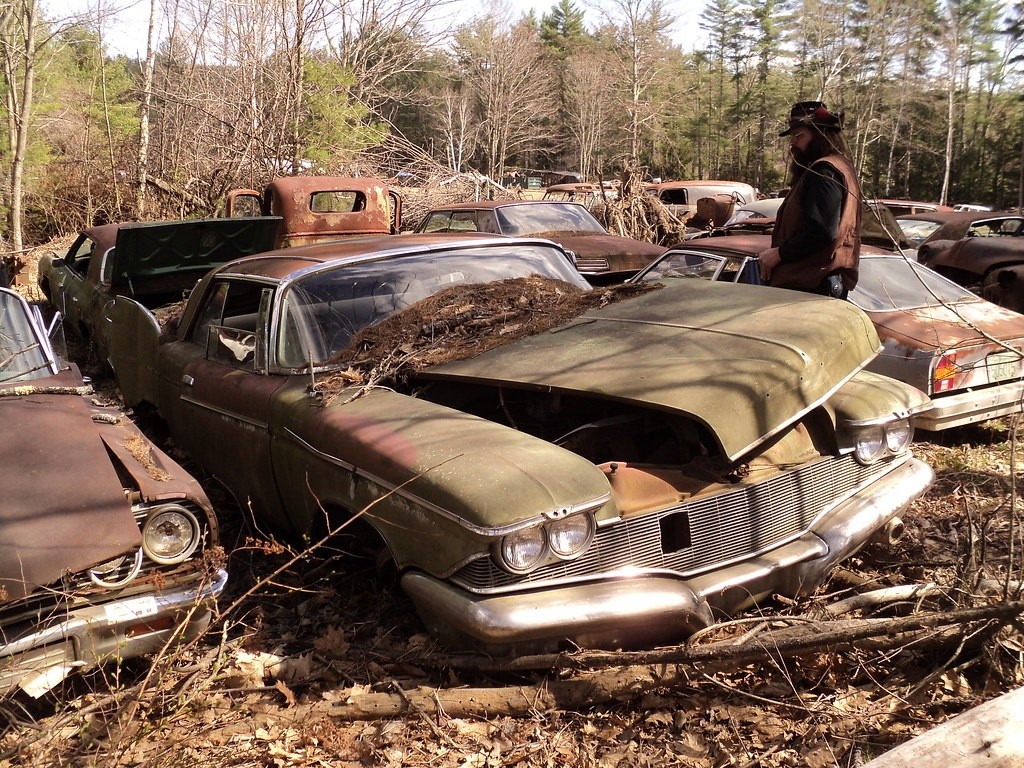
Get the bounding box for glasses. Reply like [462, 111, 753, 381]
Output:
[790, 129, 808, 139]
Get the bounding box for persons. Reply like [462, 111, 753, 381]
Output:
[759, 101, 862, 300]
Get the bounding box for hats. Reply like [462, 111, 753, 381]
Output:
[778, 101, 844, 137]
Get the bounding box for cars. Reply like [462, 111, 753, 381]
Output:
[892, 214, 978, 246]
[643, 180, 756, 226]
[109, 229, 938, 648]
[1, 285, 228, 703]
[227, 174, 403, 251]
[402, 200, 673, 277]
[953, 203, 991, 212]
[629, 233, 1023, 434]
[919, 212, 1024, 312]
[689, 197, 785, 240]
[864, 197, 954, 218]
[34, 216, 287, 363]
[543, 181, 620, 210]
